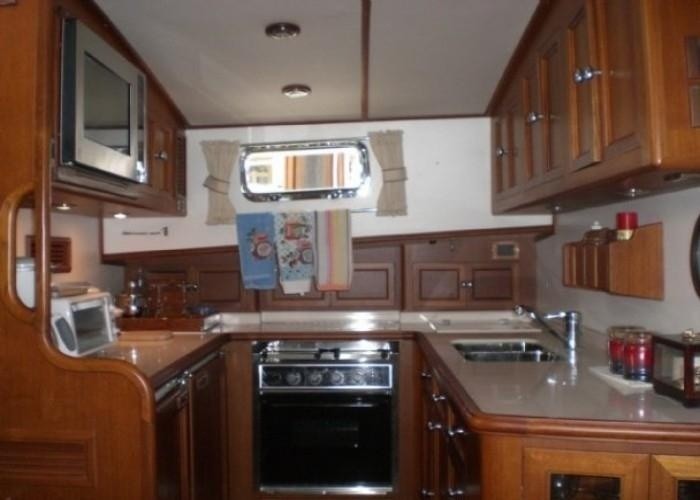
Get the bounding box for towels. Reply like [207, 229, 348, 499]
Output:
[232, 208, 355, 296]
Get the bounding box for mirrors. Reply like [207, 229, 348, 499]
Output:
[239, 142, 369, 201]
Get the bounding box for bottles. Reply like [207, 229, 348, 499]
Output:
[607, 323, 655, 384]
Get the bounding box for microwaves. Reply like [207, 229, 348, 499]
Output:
[49, 290, 119, 359]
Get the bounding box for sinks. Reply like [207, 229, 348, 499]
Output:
[450, 338, 543, 351]
[461, 350, 564, 362]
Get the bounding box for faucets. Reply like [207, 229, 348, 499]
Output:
[514, 363, 555, 403]
[516, 302, 580, 350]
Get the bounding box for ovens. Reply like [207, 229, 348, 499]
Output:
[252, 362, 398, 496]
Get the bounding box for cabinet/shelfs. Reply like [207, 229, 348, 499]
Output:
[147, 86, 188, 218]
[152, 352, 225, 499]
[490, 0, 699, 215]
[416, 344, 700, 500]
